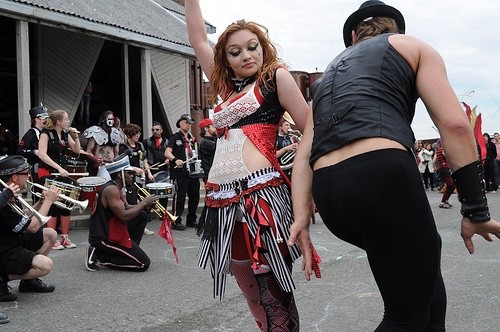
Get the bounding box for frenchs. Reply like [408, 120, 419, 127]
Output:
[280, 110, 303, 165]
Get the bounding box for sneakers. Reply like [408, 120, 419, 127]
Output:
[85, 245, 100, 272]
[0, 278, 56, 302]
[438, 202, 453, 209]
[51, 234, 77, 250]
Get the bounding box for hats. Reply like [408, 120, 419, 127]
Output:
[0, 155, 31, 176]
[104, 153, 131, 202]
[152, 121, 160, 126]
[176, 114, 195, 128]
[29, 105, 50, 120]
[343, 0, 405, 49]
[199, 118, 213, 129]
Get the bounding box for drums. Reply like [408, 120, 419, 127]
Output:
[145, 182, 178, 221]
[187, 159, 206, 179]
[41, 178, 83, 210]
[76, 175, 108, 210]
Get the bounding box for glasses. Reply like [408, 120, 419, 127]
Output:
[151, 129, 159, 132]
[126, 171, 132, 176]
[15, 169, 31, 176]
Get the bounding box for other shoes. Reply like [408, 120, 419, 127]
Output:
[144, 227, 155, 235]
[171, 221, 199, 230]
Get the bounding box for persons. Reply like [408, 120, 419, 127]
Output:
[410, 131, 500, 208]
[274, 118, 300, 182]
[182, 0, 323, 332]
[0, 104, 219, 325]
[310, 0, 500, 332]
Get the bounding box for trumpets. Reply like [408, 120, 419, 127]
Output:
[0, 179, 52, 228]
[25, 179, 89, 215]
[133, 181, 180, 223]
